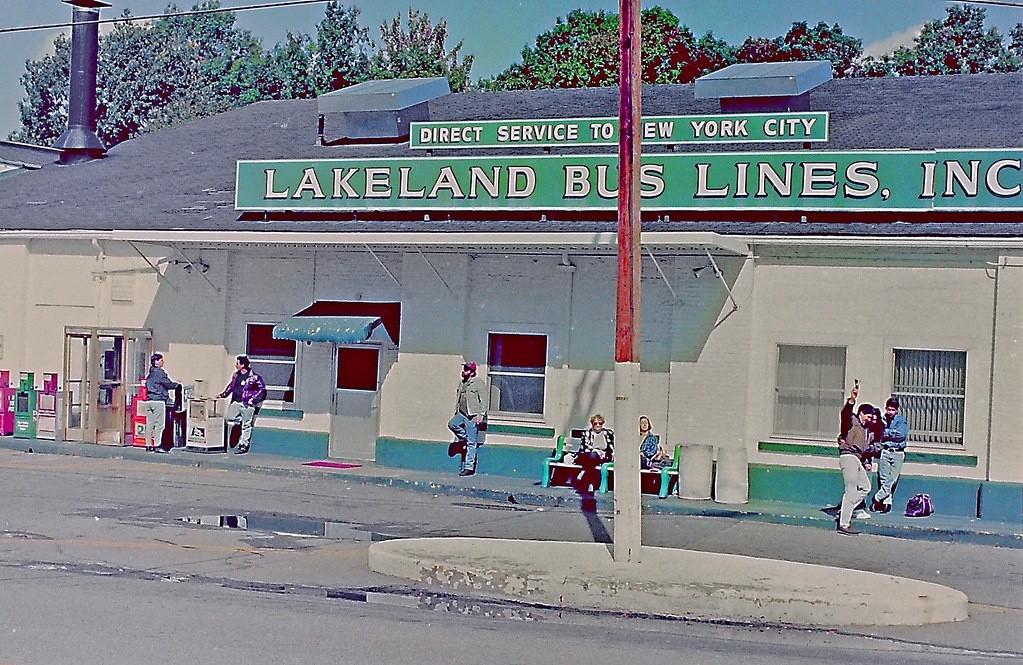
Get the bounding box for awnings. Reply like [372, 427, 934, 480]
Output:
[272, 316, 380, 342]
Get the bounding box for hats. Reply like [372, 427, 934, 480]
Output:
[461, 361, 477, 373]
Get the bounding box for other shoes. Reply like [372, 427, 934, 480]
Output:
[853, 510, 871, 519]
[235, 445, 250, 454]
[837, 526, 861, 536]
[587, 484, 594, 492]
[577, 470, 585, 480]
[880, 504, 892, 514]
[870, 497, 879, 511]
[146, 446, 155, 451]
[154, 447, 167, 453]
[458, 469, 475, 476]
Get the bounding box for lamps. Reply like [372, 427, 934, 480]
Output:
[694, 259, 724, 282]
[172, 257, 209, 274]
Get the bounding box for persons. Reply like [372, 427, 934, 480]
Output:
[837, 387, 908, 536]
[217, 356, 265, 455]
[577, 414, 614, 495]
[145, 354, 180, 454]
[449, 361, 486, 476]
[640, 416, 658, 470]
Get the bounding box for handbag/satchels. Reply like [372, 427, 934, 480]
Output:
[905, 493, 934, 516]
[651, 453, 672, 469]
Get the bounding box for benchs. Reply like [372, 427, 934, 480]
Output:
[541, 432, 682, 493]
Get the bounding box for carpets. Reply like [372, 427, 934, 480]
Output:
[302, 461, 362, 468]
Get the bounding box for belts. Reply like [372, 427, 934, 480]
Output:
[883, 446, 904, 452]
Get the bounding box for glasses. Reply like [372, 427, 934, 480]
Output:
[592, 421, 604, 425]
